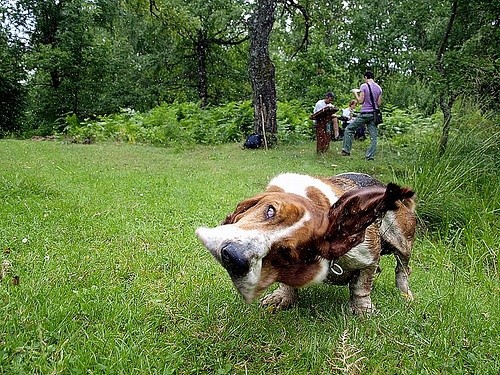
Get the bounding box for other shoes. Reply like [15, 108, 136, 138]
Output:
[363, 157, 374, 160]
[337, 150, 350, 156]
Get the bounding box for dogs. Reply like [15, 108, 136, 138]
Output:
[194, 174, 417, 318]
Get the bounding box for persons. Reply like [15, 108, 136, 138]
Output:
[338, 71, 384, 162]
[314, 92, 334, 155]
[343, 99, 367, 143]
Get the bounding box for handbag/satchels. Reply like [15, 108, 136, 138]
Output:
[375, 109, 383, 126]
